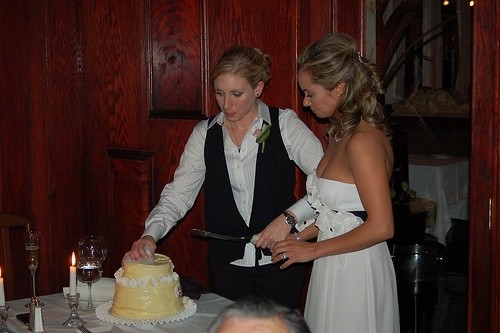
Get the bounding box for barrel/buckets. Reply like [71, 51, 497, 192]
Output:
[392, 242, 448, 333]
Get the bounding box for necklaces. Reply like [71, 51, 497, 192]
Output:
[230, 122, 240, 152]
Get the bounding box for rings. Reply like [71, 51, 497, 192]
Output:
[282, 253, 286, 260]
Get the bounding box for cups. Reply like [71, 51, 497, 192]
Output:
[78, 235, 107, 262]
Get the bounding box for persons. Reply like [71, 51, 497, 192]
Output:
[271, 34, 401, 333]
[129, 46, 325, 315]
[207, 295, 311, 333]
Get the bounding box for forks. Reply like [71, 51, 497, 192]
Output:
[78, 325, 93, 333]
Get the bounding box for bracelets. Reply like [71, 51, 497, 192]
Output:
[294, 233, 300, 241]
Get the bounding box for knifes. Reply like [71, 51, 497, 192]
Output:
[190, 228, 260, 244]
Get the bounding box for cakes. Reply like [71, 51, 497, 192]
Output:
[110, 247, 185, 320]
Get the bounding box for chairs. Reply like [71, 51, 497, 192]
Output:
[407, 244, 468, 333]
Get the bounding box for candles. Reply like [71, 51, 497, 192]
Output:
[70, 251, 76, 296]
[144, 245, 151, 257]
[0, 267, 6, 307]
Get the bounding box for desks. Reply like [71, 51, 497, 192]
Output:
[6, 293, 236, 333]
[408, 153, 470, 247]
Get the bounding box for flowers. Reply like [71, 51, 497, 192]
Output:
[253, 124, 271, 153]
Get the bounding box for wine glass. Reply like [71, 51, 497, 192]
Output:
[24, 231, 45, 309]
[75, 255, 103, 311]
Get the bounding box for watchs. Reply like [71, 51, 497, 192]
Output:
[283, 212, 296, 227]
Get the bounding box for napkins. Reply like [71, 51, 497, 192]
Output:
[63, 277, 115, 300]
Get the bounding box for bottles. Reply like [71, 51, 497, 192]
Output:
[28, 296, 36, 330]
[32, 300, 45, 333]
